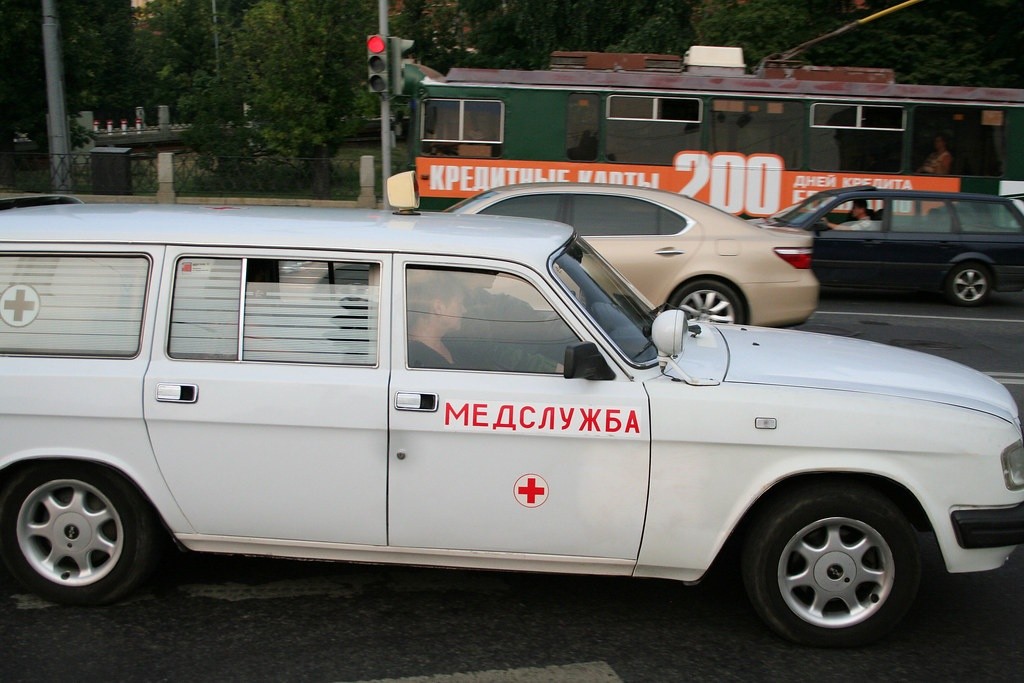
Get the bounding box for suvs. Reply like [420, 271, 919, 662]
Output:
[731, 186, 1024, 309]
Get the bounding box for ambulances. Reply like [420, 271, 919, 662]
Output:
[0, 170, 1024, 654]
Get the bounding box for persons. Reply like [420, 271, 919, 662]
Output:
[924, 133, 950, 178]
[820, 198, 875, 231]
[405, 270, 488, 371]
[445, 268, 566, 374]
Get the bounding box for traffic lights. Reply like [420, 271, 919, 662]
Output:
[367, 34, 390, 96]
[390, 37, 422, 101]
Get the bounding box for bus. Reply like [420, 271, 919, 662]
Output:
[401, 45, 1024, 226]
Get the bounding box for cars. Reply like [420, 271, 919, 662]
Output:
[320, 181, 822, 332]
